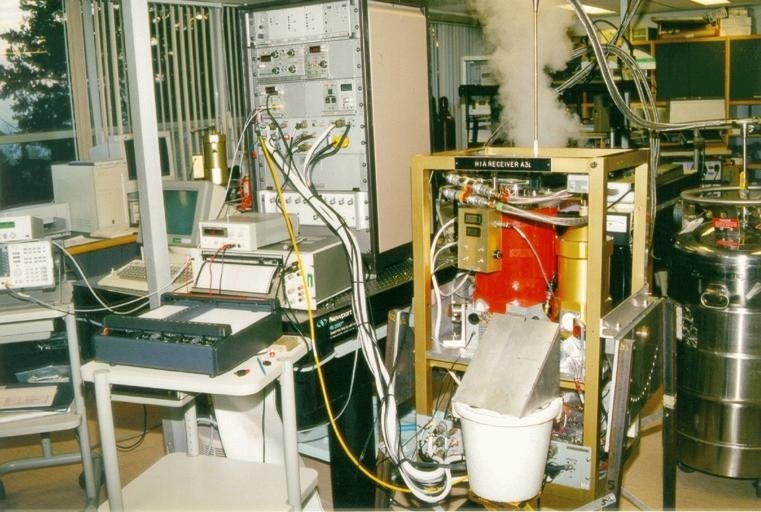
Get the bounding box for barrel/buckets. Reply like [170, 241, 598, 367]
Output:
[451, 397, 564, 503]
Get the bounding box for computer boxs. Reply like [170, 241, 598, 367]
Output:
[48, 160, 130, 232]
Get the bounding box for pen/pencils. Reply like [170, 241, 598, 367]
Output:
[257, 358, 265, 374]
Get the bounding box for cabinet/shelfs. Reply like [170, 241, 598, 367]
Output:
[0, 143, 662, 510]
[569, 33, 760, 189]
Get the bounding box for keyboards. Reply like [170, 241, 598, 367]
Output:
[97, 259, 191, 289]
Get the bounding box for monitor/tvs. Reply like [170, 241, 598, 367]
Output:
[89, 130, 179, 194]
[137, 179, 227, 247]
